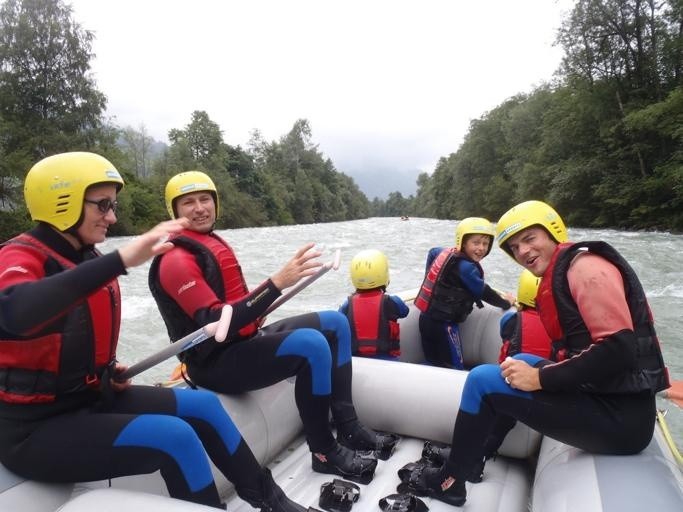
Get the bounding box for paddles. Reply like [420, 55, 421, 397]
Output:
[656, 377, 682, 408]
[167, 249, 342, 386]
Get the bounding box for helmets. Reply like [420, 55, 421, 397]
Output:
[455, 216, 496, 260]
[25, 151, 125, 233]
[517, 268, 541, 309]
[350, 251, 390, 292]
[166, 170, 220, 223]
[495, 200, 570, 246]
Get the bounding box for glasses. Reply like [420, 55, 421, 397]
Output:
[83, 196, 121, 215]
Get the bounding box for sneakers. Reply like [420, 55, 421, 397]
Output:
[310, 444, 377, 479]
[398, 466, 468, 508]
[422, 440, 487, 485]
[238, 463, 318, 512]
[336, 424, 399, 460]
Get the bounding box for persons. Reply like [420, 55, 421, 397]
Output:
[413, 216, 519, 371]
[0, 149, 324, 512]
[145, 168, 401, 485]
[336, 248, 409, 364]
[396, 198, 673, 508]
